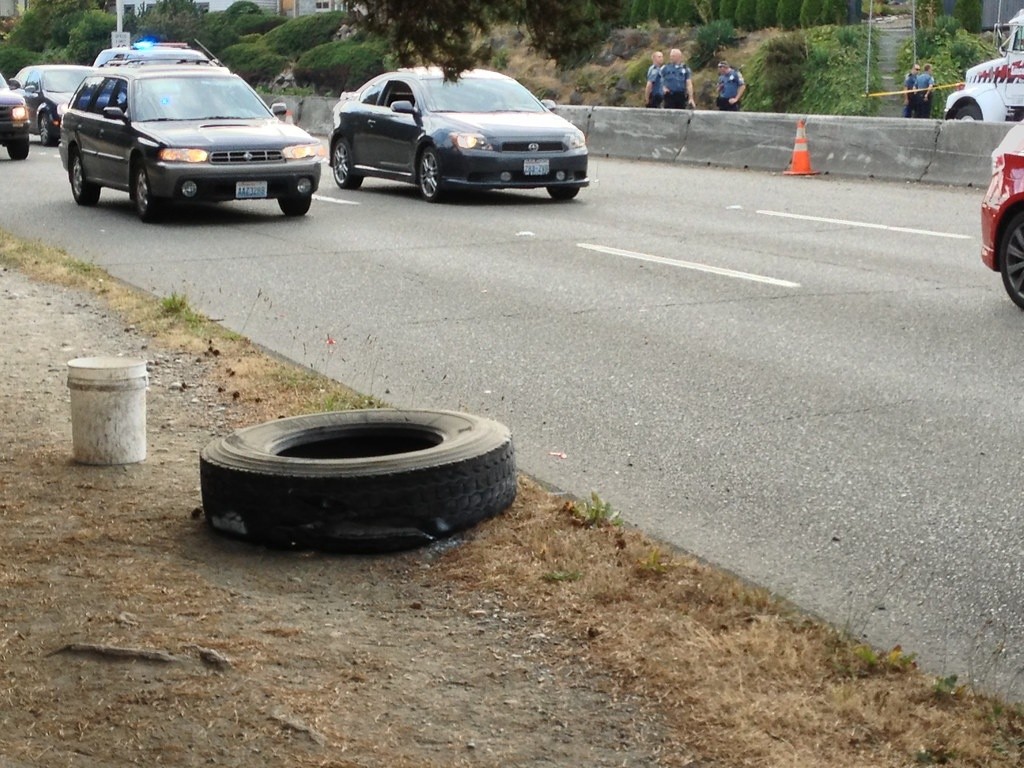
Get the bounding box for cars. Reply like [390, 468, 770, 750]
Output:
[980, 122, 1024, 310]
[90, 39, 222, 67]
[327, 66, 592, 203]
[0, 71, 32, 160]
[10, 64, 99, 146]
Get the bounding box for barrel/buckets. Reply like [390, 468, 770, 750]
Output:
[66, 356, 148, 465]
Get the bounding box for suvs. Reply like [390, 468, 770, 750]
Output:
[56, 65, 330, 224]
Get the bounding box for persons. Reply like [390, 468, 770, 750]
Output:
[715, 61, 746, 111]
[903, 63, 934, 118]
[645, 48, 697, 110]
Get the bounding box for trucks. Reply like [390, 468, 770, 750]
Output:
[940, 7, 1024, 121]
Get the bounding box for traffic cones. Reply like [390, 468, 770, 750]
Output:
[285, 111, 295, 124]
[783, 121, 820, 176]
